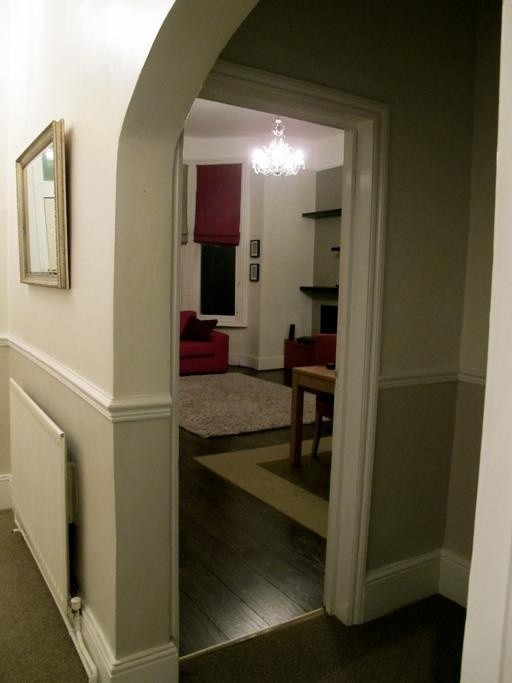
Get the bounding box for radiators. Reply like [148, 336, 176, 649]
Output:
[9, 378, 71, 618]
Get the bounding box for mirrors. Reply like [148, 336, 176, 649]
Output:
[15, 119, 69, 290]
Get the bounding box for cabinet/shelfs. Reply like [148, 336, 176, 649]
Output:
[300, 209, 342, 301]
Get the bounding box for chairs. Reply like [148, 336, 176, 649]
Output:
[180, 311, 229, 374]
[283, 333, 336, 371]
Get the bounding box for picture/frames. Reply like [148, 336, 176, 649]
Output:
[249, 240, 259, 257]
[249, 263, 259, 281]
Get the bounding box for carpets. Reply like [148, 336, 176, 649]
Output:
[179, 372, 332, 442]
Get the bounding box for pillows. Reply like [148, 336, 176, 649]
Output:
[180, 318, 218, 341]
[296, 335, 314, 343]
